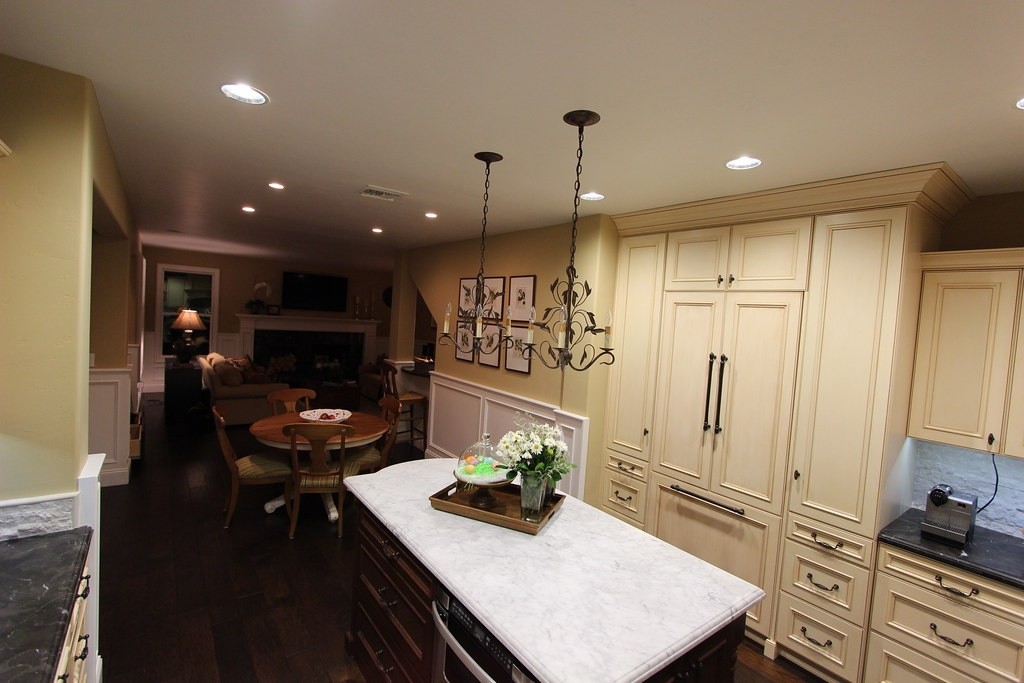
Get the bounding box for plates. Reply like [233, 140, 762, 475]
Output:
[299, 409, 352, 424]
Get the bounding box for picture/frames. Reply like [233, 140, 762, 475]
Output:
[454, 275, 537, 374]
[267, 305, 280, 315]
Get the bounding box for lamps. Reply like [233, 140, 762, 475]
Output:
[171, 308, 207, 362]
[438, 152, 515, 353]
[521, 109, 616, 371]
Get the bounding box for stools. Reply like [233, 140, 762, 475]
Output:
[375, 363, 427, 456]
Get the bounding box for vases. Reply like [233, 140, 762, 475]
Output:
[519, 475, 548, 522]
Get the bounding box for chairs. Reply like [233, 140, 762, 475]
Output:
[212, 389, 402, 542]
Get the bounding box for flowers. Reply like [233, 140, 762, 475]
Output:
[495, 409, 576, 514]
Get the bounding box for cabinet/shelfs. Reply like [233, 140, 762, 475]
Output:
[165, 363, 212, 436]
[53, 556, 91, 683]
[906, 247, 1024, 460]
[597, 204, 961, 683]
[344, 494, 439, 683]
[862, 540, 1024, 683]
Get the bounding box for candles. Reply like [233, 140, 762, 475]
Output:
[356, 296, 360, 304]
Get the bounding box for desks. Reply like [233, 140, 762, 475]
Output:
[249, 410, 390, 522]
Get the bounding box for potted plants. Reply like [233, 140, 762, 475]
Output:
[245, 299, 264, 314]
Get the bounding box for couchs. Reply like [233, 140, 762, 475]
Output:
[357, 364, 381, 400]
[195, 354, 289, 425]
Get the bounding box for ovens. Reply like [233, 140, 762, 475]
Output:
[431, 587, 540, 683]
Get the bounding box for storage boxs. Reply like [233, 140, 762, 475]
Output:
[130, 410, 143, 459]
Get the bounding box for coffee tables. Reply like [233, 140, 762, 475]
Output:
[304, 378, 359, 410]
